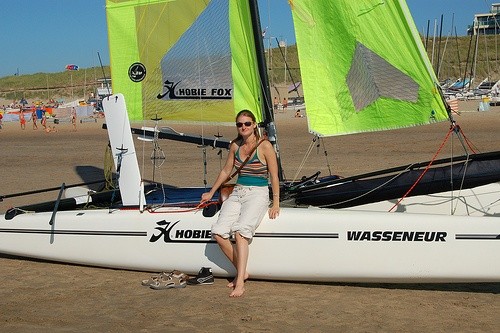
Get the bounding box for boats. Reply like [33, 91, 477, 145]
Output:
[0, 10, 500, 118]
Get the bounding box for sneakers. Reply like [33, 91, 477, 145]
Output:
[187, 268, 215, 285]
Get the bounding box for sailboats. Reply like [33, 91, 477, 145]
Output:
[0, 0, 500, 284]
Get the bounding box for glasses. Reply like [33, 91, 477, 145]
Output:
[235, 122, 252, 128]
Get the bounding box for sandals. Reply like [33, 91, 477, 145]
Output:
[141, 269, 188, 290]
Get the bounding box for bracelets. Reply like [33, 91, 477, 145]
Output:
[273, 193, 280, 198]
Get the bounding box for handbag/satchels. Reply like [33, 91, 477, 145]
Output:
[219, 183, 238, 202]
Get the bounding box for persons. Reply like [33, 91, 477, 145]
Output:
[274, 96, 301, 117]
[200, 109, 280, 296]
[0, 98, 77, 133]
